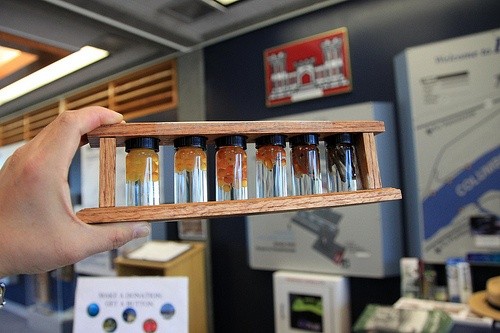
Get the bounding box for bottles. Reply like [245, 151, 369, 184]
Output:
[172, 135, 209, 205]
[214, 135, 248, 200]
[254, 135, 288, 199]
[285, 135, 323, 195]
[125, 136, 160, 206]
[324, 135, 357, 194]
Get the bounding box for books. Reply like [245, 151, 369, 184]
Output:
[354, 297, 494, 333]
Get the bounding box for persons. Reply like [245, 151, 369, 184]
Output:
[0, 106, 152, 281]
